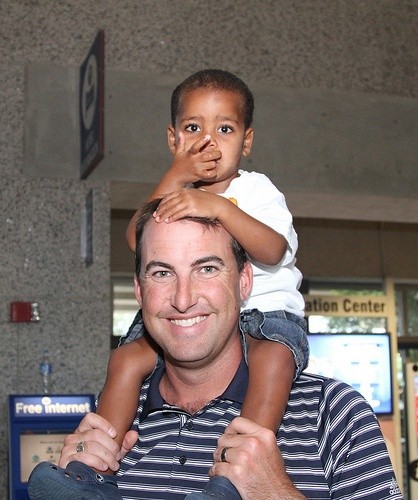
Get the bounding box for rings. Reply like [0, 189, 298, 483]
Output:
[75, 443, 84, 452]
[220, 447, 231, 462]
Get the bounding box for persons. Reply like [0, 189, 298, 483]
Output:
[26, 69, 404, 500]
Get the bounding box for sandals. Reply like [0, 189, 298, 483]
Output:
[26, 460, 123, 500]
[184, 475, 242, 500]
